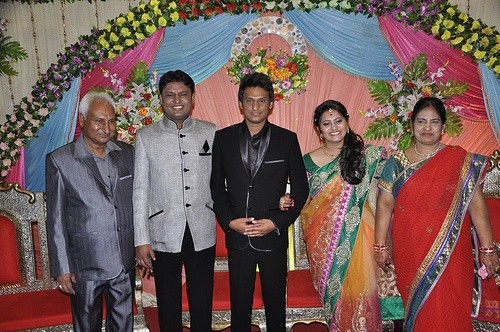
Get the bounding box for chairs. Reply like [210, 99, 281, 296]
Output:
[0, 149, 500, 332]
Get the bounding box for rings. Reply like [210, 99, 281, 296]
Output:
[58, 285, 62, 289]
[140, 261, 143, 263]
[279, 197, 285, 209]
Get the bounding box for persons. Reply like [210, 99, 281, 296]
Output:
[373, 96, 500, 332]
[132, 69, 222, 332]
[210, 72, 309, 332]
[279, 100, 405, 332]
[45, 90, 152, 332]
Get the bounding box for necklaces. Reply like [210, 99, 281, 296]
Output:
[323, 145, 341, 160]
[414, 143, 442, 157]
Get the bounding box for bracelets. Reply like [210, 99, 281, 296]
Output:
[479, 246, 497, 255]
[374, 244, 388, 252]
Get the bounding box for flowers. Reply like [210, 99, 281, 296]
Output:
[358, 51, 471, 154]
[88, 61, 165, 147]
[223, 46, 312, 104]
[0, 0, 500, 186]
